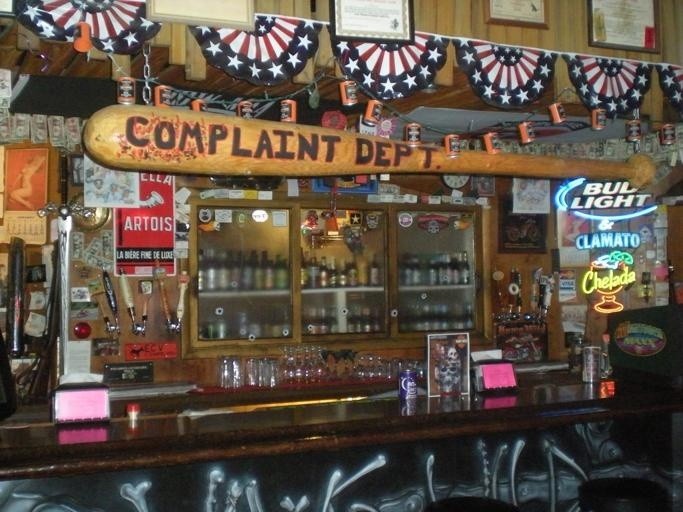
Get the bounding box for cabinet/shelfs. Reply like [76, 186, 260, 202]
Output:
[180, 193, 493, 362]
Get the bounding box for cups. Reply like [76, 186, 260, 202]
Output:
[215, 356, 278, 388]
[397, 369, 417, 398]
[399, 398, 416, 416]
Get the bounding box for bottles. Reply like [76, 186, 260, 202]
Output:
[197, 301, 291, 339]
[397, 300, 474, 331]
[300, 304, 381, 334]
[197, 249, 288, 292]
[299, 246, 381, 288]
[396, 250, 471, 285]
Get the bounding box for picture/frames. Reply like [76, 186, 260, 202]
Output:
[328, 0, 415, 45]
[481, 0, 549, 30]
[586, 0, 661, 54]
[147, 0, 257, 32]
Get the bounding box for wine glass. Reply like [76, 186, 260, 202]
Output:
[279, 343, 357, 383]
[356, 355, 427, 383]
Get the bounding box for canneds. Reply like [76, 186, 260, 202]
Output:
[339, 80, 359, 106]
[154, 85, 171, 107]
[406, 101, 676, 159]
[280, 99, 297, 122]
[191, 99, 206, 111]
[73, 21, 91, 52]
[237, 100, 254, 119]
[116, 76, 136, 104]
[399, 369, 417, 400]
[399, 400, 416, 419]
[364, 99, 383, 126]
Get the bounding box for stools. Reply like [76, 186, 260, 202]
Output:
[423, 495, 519, 512]
[578, 477, 672, 512]
